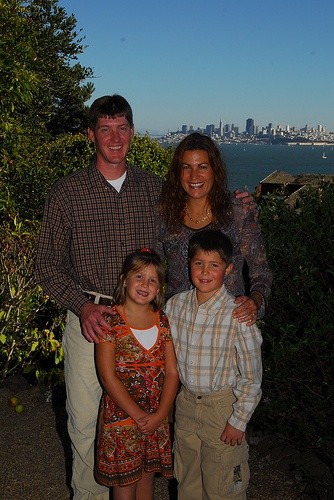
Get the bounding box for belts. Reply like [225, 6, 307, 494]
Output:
[83, 292, 115, 307]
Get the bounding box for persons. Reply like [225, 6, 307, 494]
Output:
[40, 95, 257, 500]
[153, 132, 273, 500]
[163, 230, 263, 500]
[93, 247, 180, 500]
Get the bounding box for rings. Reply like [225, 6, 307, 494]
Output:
[251, 313, 255, 316]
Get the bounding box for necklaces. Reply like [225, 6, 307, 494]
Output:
[185, 195, 212, 224]
